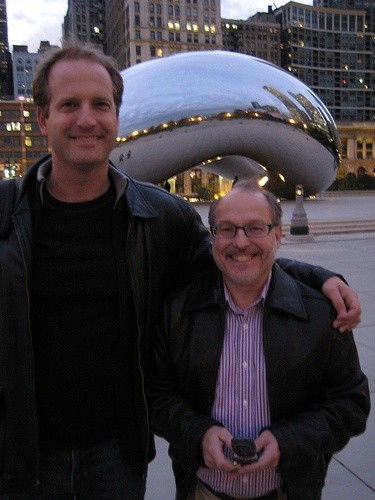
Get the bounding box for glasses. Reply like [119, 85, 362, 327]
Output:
[209, 222, 279, 240]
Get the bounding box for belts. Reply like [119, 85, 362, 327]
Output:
[199, 478, 287, 500]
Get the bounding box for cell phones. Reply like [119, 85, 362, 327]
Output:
[231, 437, 259, 466]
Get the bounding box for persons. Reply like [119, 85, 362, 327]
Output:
[0, 37, 362, 500]
[141, 175, 371, 500]
